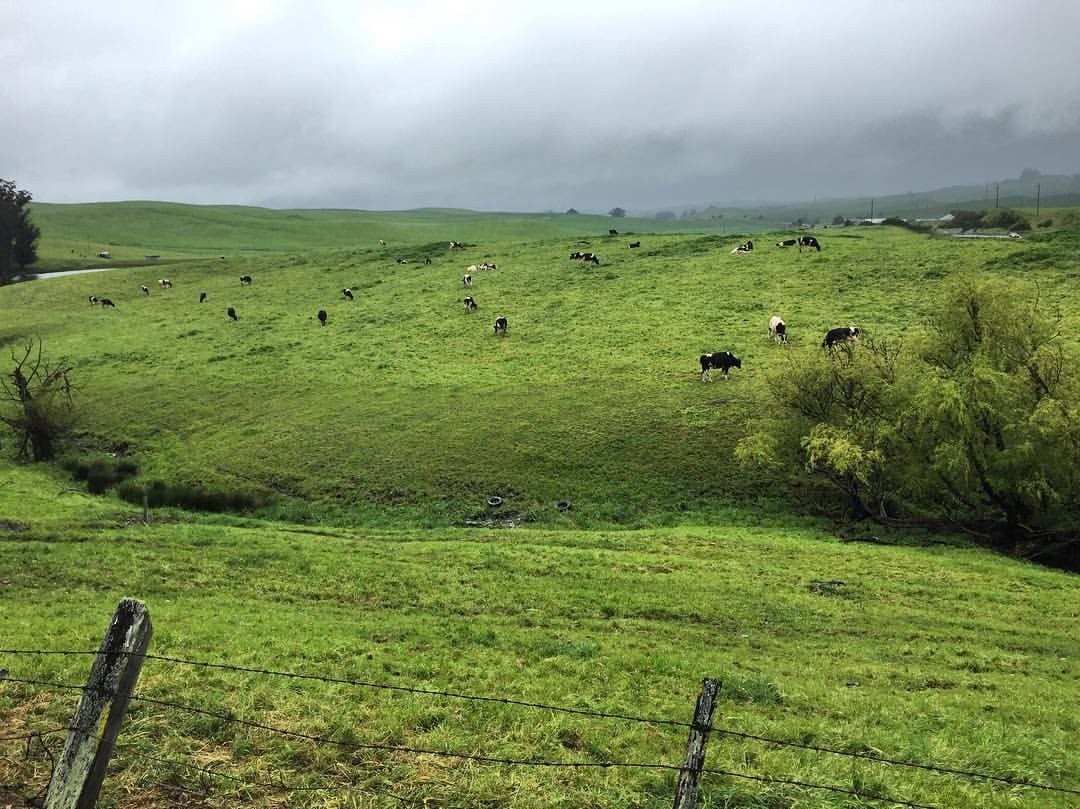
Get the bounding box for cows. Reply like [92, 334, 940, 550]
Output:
[463, 274, 472, 289]
[449, 240, 465, 250]
[157, 278, 173, 290]
[822, 326, 864, 349]
[699, 351, 741, 383]
[767, 315, 788, 345]
[137, 284, 152, 297]
[466, 262, 497, 273]
[463, 296, 478, 314]
[492, 316, 508, 335]
[627, 241, 641, 249]
[198, 291, 208, 303]
[608, 229, 618, 236]
[343, 286, 354, 300]
[568, 251, 600, 266]
[317, 310, 329, 328]
[775, 236, 821, 253]
[226, 307, 238, 321]
[88, 296, 115, 310]
[239, 275, 253, 287]
[730, 240, 753, 255]
